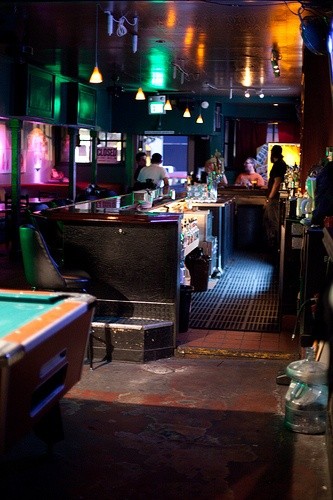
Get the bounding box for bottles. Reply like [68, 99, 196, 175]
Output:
[282, 346, 331, 433]
[181, 216, 200, 248]
[281, 178, 297, 189]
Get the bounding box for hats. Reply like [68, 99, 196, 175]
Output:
[206, 158, 221, 163]
[215, 151, 221, 155]
[272, 145, 284, 158]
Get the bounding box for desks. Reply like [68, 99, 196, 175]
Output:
[0, 288, 96, 500]
[0, 196, 56, 217]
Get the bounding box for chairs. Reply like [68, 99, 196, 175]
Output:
[19, 223, 94, 370]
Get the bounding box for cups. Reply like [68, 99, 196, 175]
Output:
[251, 177, 258, 189]
[186, 184, 217, 200]
[296, 197, 307, 217]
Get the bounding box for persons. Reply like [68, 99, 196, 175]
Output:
[52, 166, 69, 183]
[233, 157, 265, 187]
[264, 145, 287, 232]
[125, 152, 147, 193]
[136, 153, 168, 190]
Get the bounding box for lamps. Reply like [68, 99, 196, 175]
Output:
[183, 80, 191, 117]
[271, 49, 280, 77]
[135, 48, 146, 100]
[89, 0, 103, 83]
[255, 88, 264, 98]
[163, 65, 172, 111]
[241, 88, 250, 98]
[196, 83, 204, 124]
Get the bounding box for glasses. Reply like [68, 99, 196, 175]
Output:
[244, 161, 253, 164]
